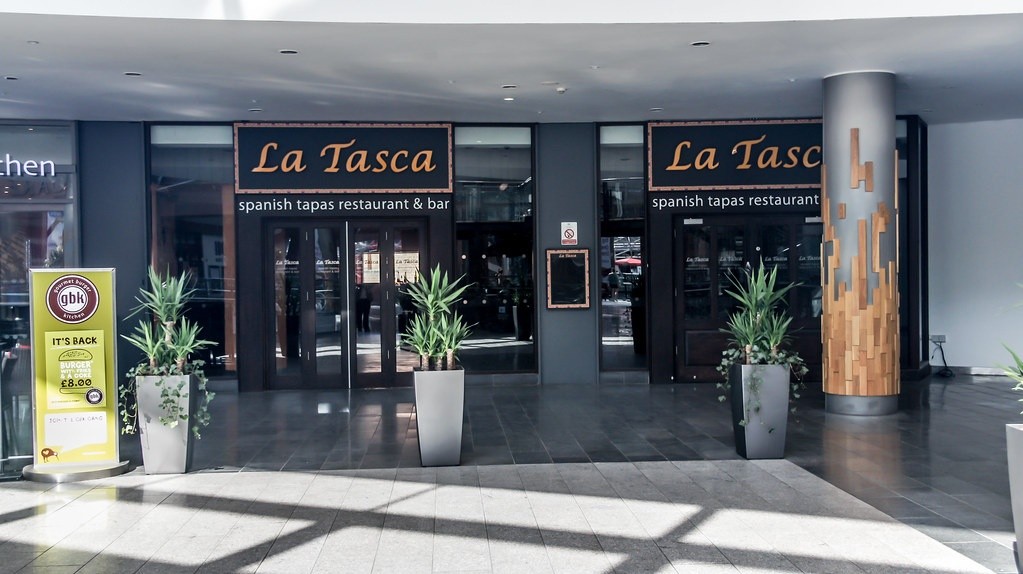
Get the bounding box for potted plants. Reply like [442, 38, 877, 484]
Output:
[119, 261, 221, 472]
[396, 262, 480, 468]
[993, 343, 1023, 574]
[716, 253, 809, 460]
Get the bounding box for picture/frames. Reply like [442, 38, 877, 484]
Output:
[545, 247, 591, 310]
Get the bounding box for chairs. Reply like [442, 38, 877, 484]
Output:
[0, 353, 18, 476]
